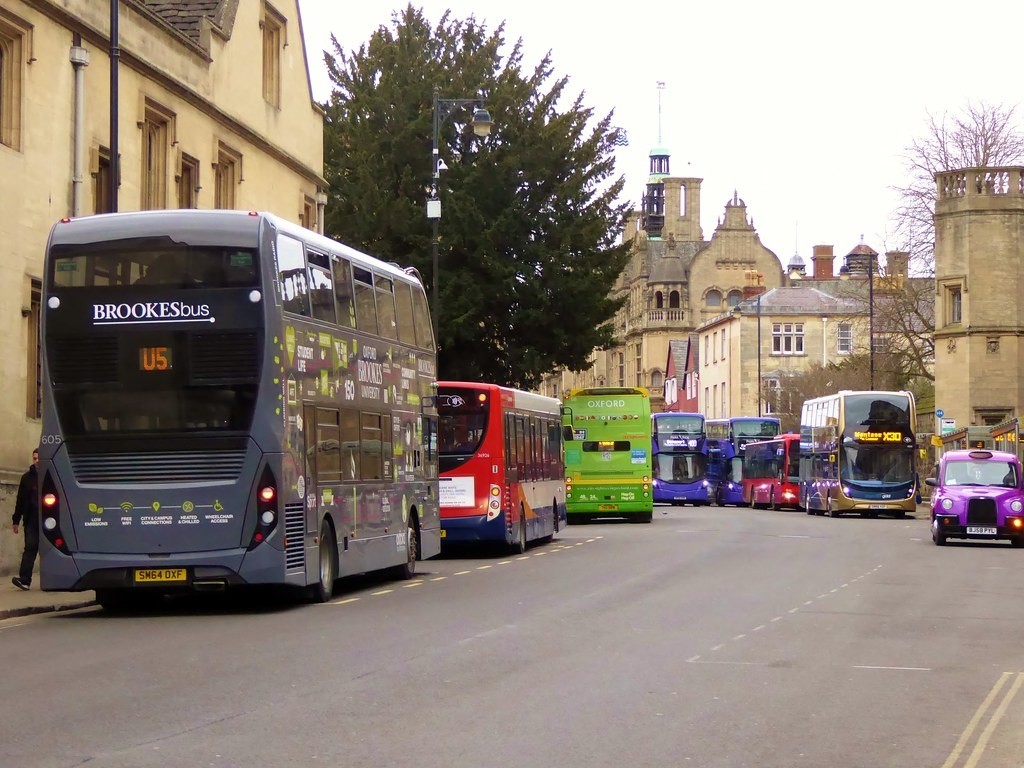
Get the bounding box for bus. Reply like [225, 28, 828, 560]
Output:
[650, 411, 708, 507]
[432, 379, 565, 554]
[561, 387, 656, 523]
[798, 389, 917, 519]
[742, 433, 800, 512]
[705, 416, 782, 507]
[35, 206, 441, 605]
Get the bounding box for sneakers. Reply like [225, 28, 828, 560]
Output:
[12, 577, 30, 590]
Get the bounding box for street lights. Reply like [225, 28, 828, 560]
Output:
[840, 252, 874, 391]
[733, 294, 762, 417]
[429, 83, 496, 348]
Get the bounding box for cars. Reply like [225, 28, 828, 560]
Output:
[922, 448, 1024, 548]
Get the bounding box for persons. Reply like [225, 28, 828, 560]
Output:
[11, 449, 42, 591]
